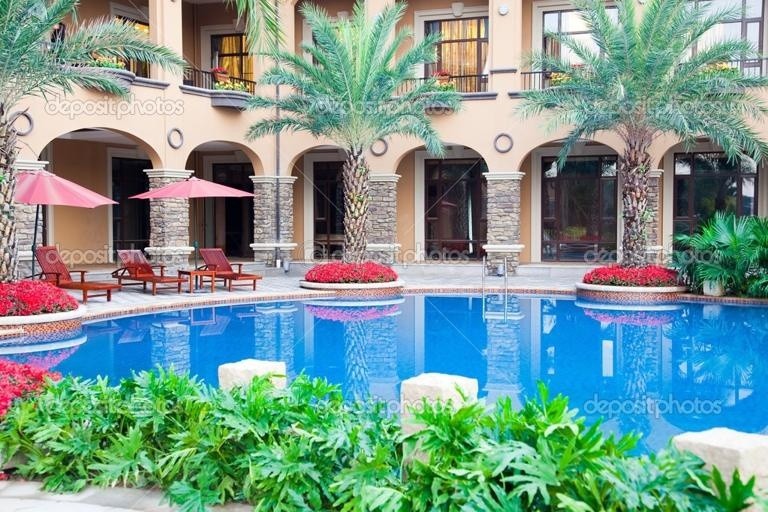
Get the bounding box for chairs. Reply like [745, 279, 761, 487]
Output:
[24, 244, 124, 303]
[79, 303, 267, 349]
[195, 245, 263, 293]
[109, 246, 190, 297]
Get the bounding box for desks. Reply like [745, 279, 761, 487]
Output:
[175, 267, 217, 295]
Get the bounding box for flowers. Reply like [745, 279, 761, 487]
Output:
[701, 56, 739, 80]
[426, 68, 459, 92]
[548, 69, 574, 88]
[209, 64, 253, 92]
[72, 55, 128, 71]
